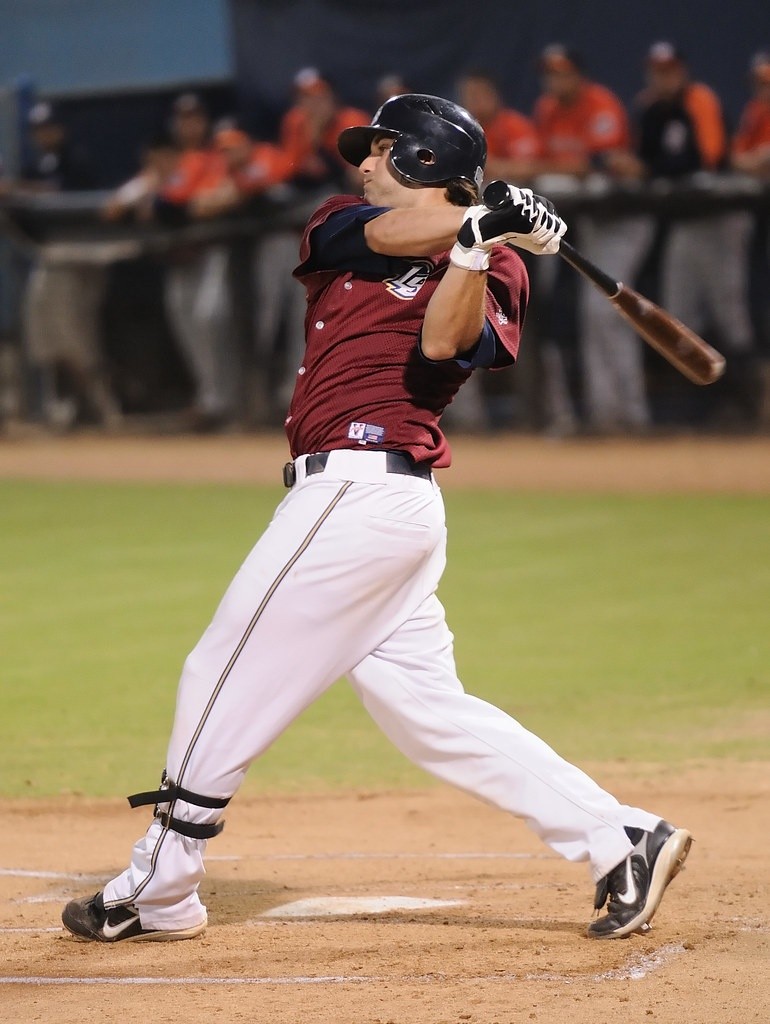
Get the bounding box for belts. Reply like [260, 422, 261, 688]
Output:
[283, 450, 431, 487]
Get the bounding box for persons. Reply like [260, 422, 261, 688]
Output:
[0, 40, 770, 441]
[61, 93, 692, 941]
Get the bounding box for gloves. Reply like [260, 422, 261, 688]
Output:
[449, 185, 568, 271]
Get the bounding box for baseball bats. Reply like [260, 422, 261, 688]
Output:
[478, 174, 730, 384]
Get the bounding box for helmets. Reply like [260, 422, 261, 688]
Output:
[336, 93, 488, 192]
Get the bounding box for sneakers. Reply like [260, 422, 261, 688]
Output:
[62, 890, 208, 942]
[586, 820, 693, 939]
